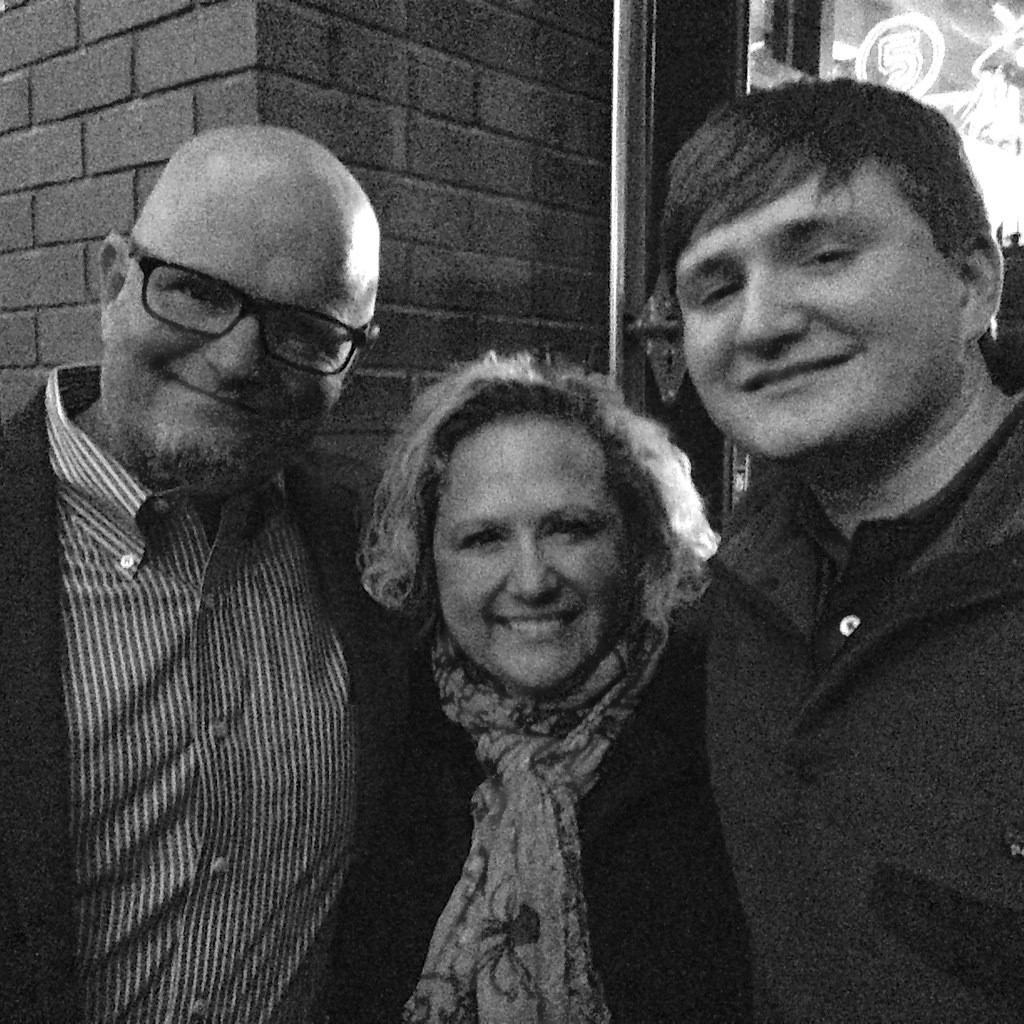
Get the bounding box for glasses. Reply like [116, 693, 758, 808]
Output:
[124, 232, 374, 378]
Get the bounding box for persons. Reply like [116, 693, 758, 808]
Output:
[335, 356, 732, 1024]
[655, 75, 1023, 1022]
[4, 119, 405, 1024]
